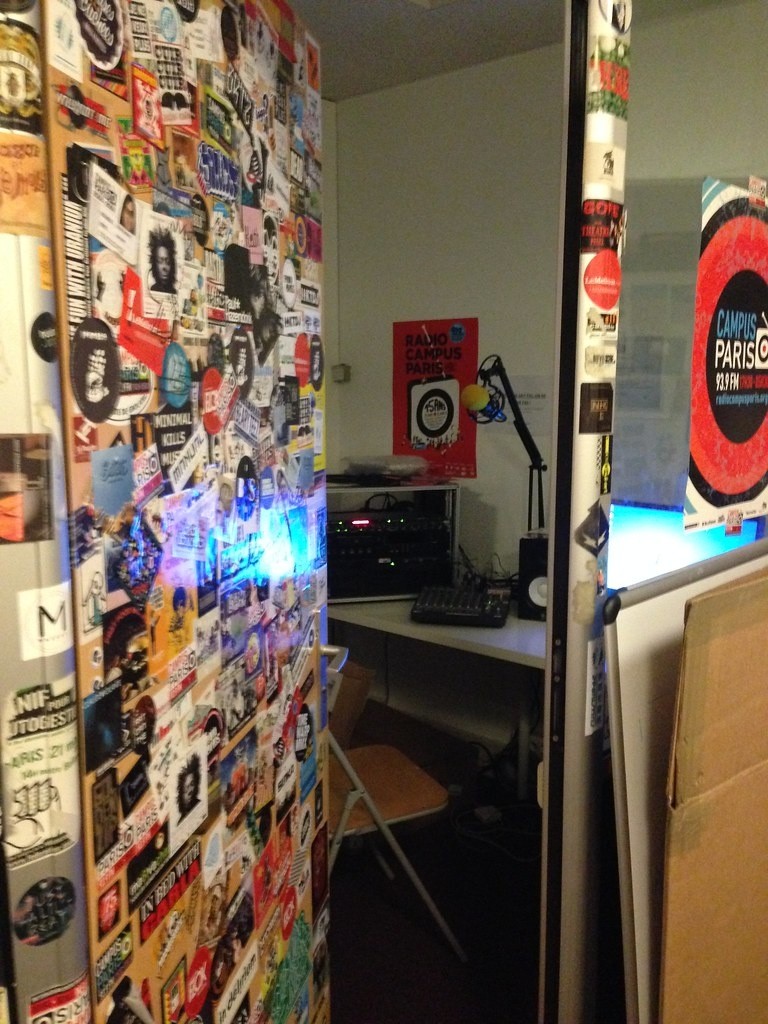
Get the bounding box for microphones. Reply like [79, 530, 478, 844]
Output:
[461, 384, 507, 423]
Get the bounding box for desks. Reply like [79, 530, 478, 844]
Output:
[326, 599, 551, 754]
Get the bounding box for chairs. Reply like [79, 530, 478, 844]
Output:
[327, 724, 471, 964]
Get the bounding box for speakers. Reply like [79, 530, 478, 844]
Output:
[517, 537, 549, 621]
